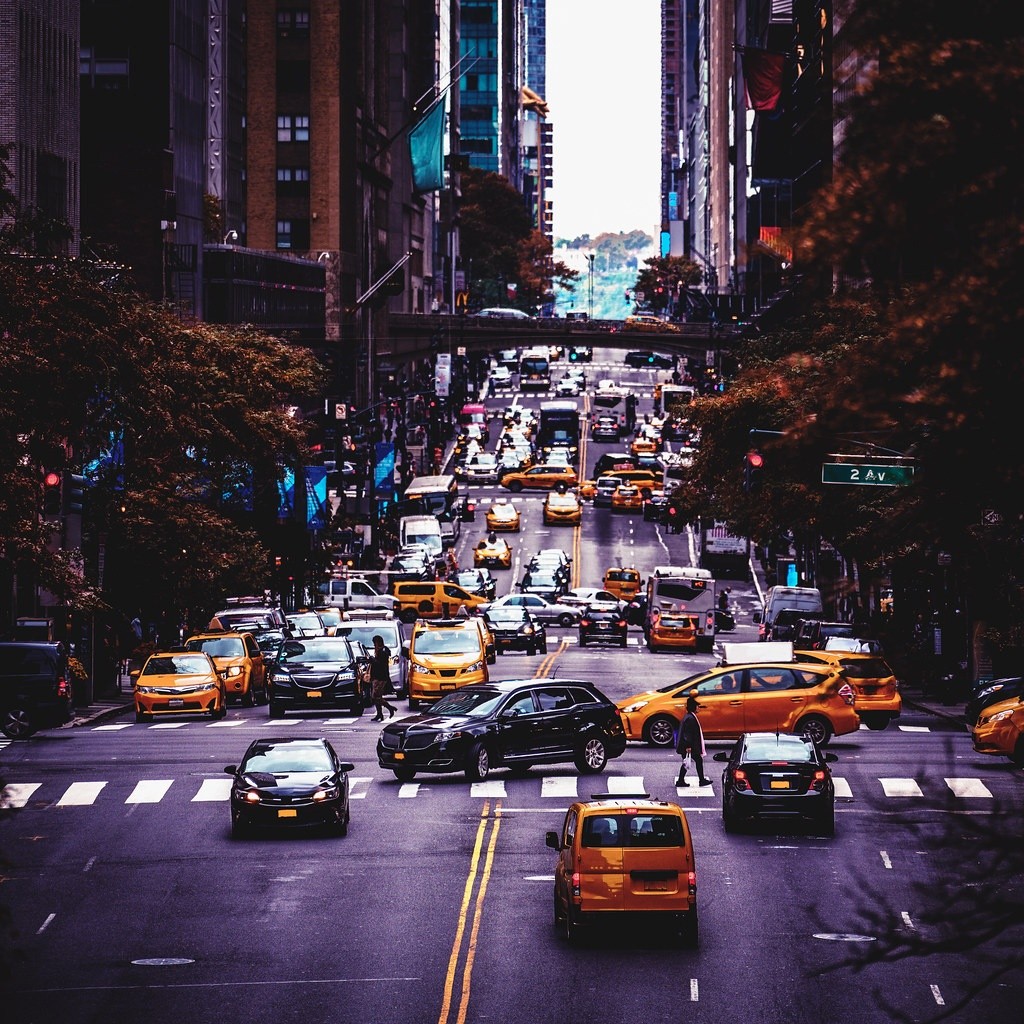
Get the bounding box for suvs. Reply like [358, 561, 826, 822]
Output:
[710, 731, 839, 835]
[615, 660, 861, 751]
[376, 680, 628, 784]
[545, 793, 698, 941]
[793, 648, 904, 731]
[0, 638, 74, 741]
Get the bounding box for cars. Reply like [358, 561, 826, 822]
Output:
[223, 735, 356, 841]
[125, 339, 890, 723]
[963, 675, 1024, 769]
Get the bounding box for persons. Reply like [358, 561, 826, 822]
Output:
[676, 698, 713, 787]
[368, 635, 398, 722]
[718, 590, 728, 610]
[721, 677, 734, 692]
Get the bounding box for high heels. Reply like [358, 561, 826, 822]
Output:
[371, 713, 384, 721]
[388, 706, 398, 718]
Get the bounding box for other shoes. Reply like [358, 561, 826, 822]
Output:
[676, 780, 690, 787]
[699, 778, 713, 786]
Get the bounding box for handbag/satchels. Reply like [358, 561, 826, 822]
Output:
[682, 753, 696, 773]
[363, 663, 372, 683]
